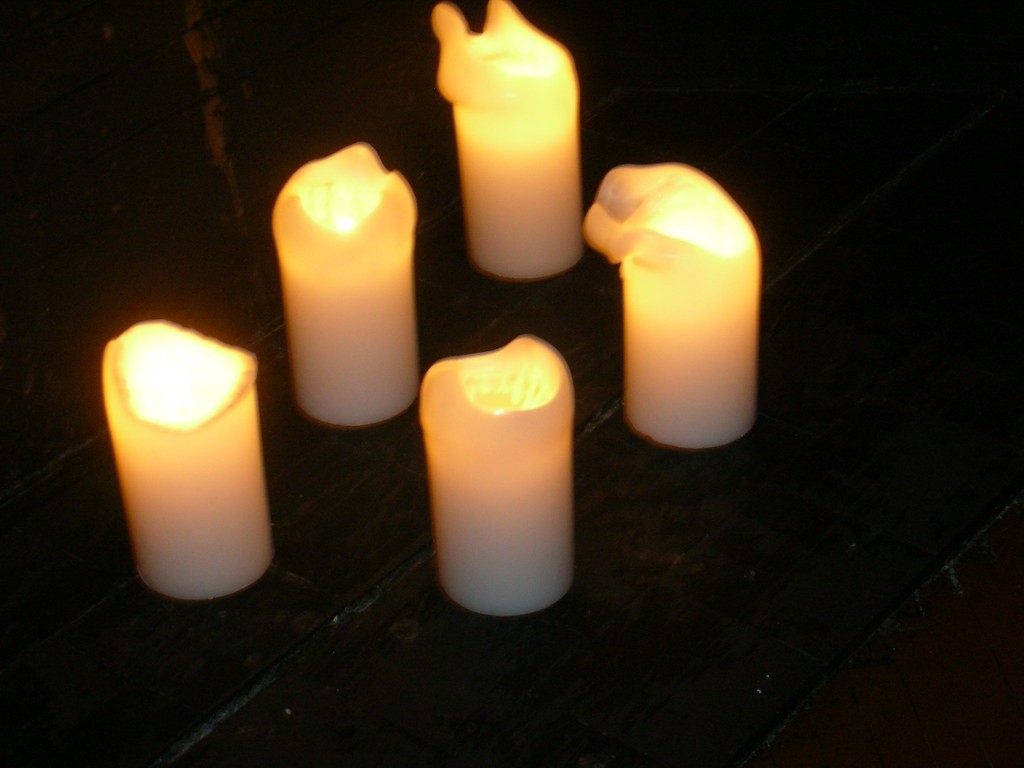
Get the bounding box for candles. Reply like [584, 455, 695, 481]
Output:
[266, 138, 420, 427]
[99, 320, 276, 601]
[424, 0, 583, 283]
[419, 332, 575, 619]
[580, 160, 762, 450]
